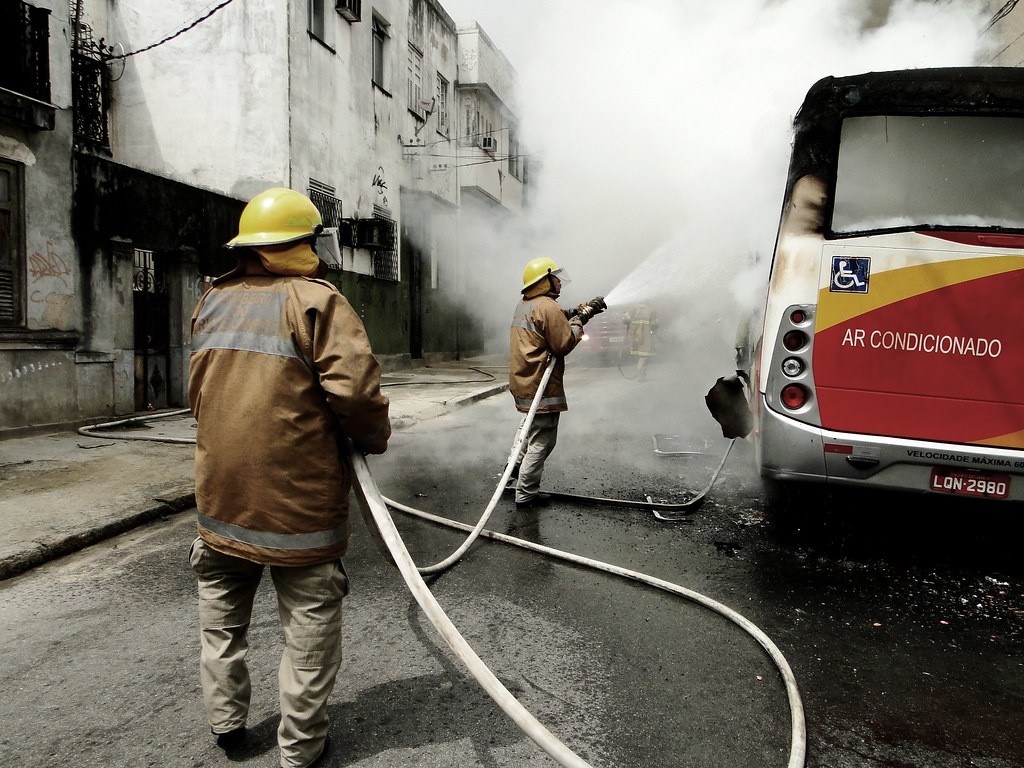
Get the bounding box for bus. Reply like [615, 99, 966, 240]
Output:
[749, 65, 1024, 508]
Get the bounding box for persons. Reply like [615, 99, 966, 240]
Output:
[188, 188, 393, 768]
[622, 296, 660, 382]
[504, 258, 608, 510]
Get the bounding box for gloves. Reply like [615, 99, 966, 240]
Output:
[588, 296, 607, 312]
[571, 309, 589, 326]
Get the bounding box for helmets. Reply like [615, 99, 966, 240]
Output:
[521, 258, 565, 294]
[222, 188, 323, 247]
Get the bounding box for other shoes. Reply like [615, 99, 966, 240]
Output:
[307, 735, 330, 768]
[217, 726, 246, 746]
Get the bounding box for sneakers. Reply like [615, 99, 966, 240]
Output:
[504, 463, 520, 480]
[516, 494, 551, 508]
